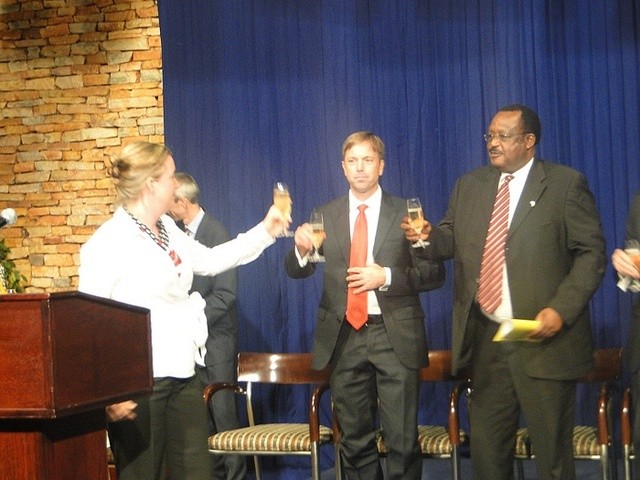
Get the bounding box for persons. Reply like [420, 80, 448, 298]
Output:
[165, 170, 250, 479]
[398, 102, 609, 479]
[73, 140, 293, 480]
[609, 191, 639, 477]
[283, 129, 446, 480]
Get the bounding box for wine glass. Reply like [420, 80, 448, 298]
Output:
[272, 182, 293, 238]
[309, 212, 326, 263]
[407, 197, 431, 248]
[623, 239, 640, 292]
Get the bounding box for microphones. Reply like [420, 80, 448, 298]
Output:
[0, 208, 16, 227]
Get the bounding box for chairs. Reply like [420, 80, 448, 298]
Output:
[622, 388, 636, 479]
[513, 348, 622, 480]
[331, 349, 470, 480]
[203, 351, 331, 480]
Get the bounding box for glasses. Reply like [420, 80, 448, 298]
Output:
[484, 133, 526, 141]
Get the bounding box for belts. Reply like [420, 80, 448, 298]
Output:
[368, 315, 383, 324]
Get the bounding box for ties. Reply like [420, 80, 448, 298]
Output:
[346, 205, 368, 331]
[477, 176, 514, 314]
